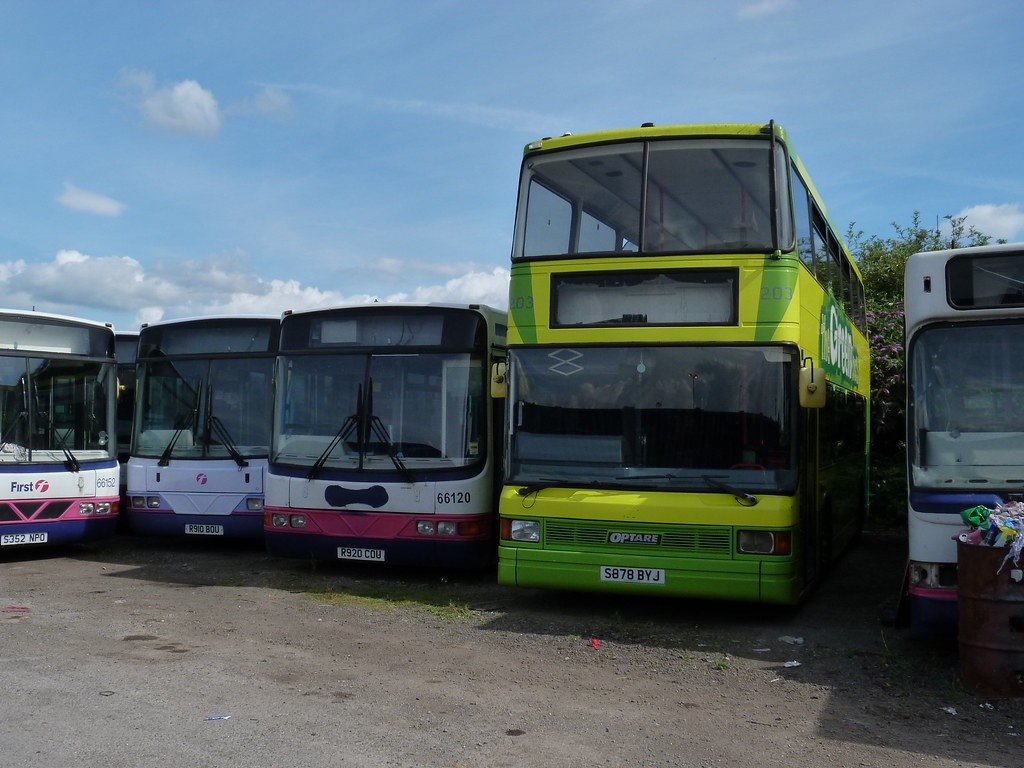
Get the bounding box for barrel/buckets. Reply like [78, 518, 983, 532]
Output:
[956, 540, 1024, 698]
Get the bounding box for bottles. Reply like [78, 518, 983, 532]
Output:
[959, 533, 968, 542]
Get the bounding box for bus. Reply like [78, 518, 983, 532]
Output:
[494, 116, 875, 611]
[261, 300, 508, 571]
[0, 305, 121, 556]
[123, 312, 279, 540]
[899, 240, 1024, 645]
[49, 328, 139, 518]
